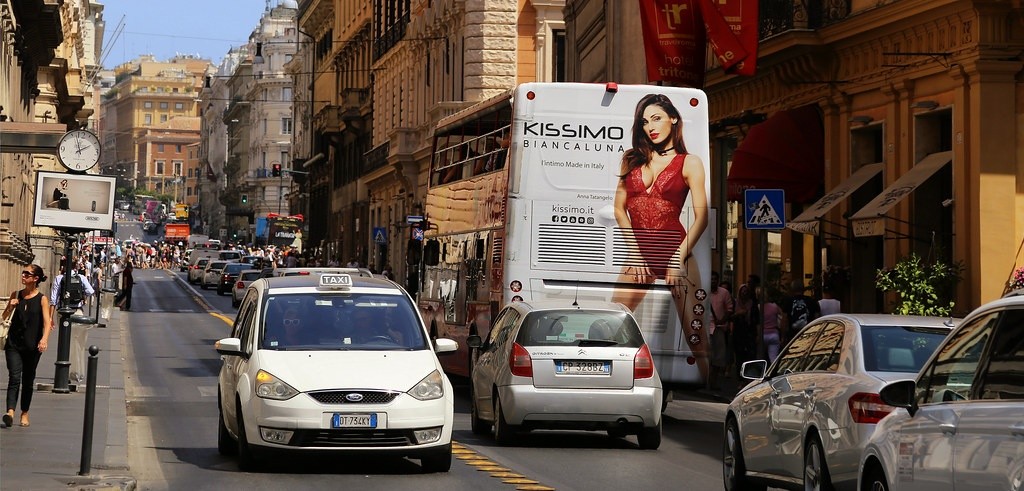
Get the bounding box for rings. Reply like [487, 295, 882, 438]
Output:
[44, 346, 45, 348]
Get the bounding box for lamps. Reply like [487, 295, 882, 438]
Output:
[942, 199, 953, 207]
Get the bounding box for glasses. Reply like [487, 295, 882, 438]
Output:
[79, 264, 82, 265]
[22, 270, 35, 277]
[283, 319, 301, 327]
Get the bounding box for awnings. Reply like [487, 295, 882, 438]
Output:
[783, 164, 884, 240]
[848, 151, 956, 245]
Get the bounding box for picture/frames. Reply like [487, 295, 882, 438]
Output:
[31, 171, 119, 232]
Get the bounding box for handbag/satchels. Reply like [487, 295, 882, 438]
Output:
[715, 318, 729, 332]
[0, 314, 12, 350]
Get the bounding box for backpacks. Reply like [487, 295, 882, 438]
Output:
[56, 274, 86, 309]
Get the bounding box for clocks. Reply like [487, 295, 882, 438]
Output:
[56, 129, 102, 173]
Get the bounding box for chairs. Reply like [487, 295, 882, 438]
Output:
[887, 348, 916, 370]
[539, 318, 624, 345]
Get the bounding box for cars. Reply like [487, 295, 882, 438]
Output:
[467, 298, 665, 450]
[721, 313, 965, 491]
[115, 193, 190, 243]
[856, 288, 1024, 491]
[182, 232, 272, 306]
[213, 272, 459, 472]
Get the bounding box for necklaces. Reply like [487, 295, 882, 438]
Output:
[656, 147, 674, 153]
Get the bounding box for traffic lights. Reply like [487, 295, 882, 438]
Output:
[273, 163, 281, 177]
[242, 194, 248, 203]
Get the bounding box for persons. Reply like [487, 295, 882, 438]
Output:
[611, 94, 709, 383]
[46, 179, 68, 208]
[719, 274, 821, 389]
[219, 240, 395, 281]
[114, 210, 143, 222]
[706, 270, 733, 396]
[59, 237, 180, 311]
[0, 264, 50, 425]
[442, 127, 510, 184]
[818, 285, 841, 316]
[49, 258, 94, 330]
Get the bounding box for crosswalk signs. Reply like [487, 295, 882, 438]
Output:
[744, 189, 786, 230]
[373, 228, 387, 244]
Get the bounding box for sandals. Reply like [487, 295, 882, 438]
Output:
[2, 413, 13, 427]
[20, 413, 30, 427]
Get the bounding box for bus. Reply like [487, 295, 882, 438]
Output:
[405, 80, 714, 411]
